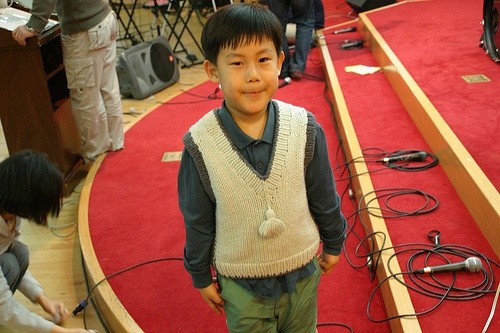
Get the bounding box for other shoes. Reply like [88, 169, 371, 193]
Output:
[279, 70, 304, 81]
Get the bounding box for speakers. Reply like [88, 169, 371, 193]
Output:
[117, 35, 179, 99]
[346, 0, 396, 12]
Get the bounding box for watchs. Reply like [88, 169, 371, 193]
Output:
[27, 25, 35, 32]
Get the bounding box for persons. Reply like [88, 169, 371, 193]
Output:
[12, 0, 124, 165]
[0, 150, 97, 333]
[177, 4, 347, 333]
[268, 0, 324, 79]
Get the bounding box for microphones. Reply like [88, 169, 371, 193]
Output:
[331, 26, 356, 34]
[376, 151, 426, 163]
[342, 40, 364, 48]
[413, 257, 482, 274]
